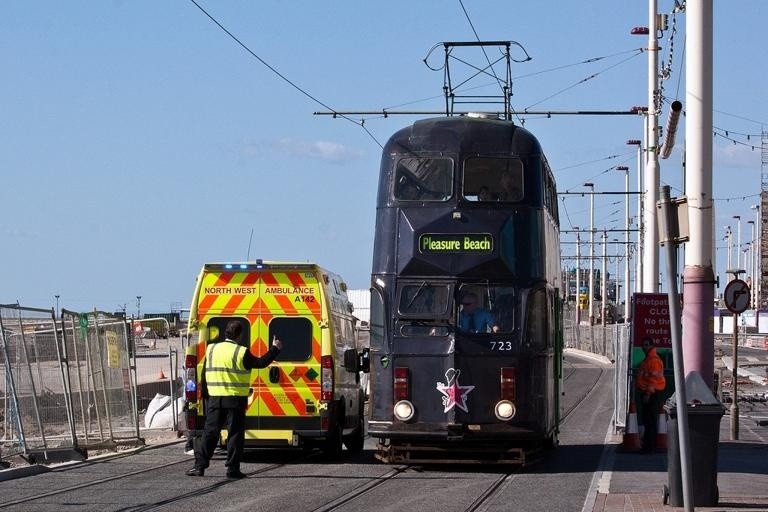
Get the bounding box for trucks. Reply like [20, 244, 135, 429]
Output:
[597, 304, 618, 324]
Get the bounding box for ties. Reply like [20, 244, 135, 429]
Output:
[468, 310, 475, 331]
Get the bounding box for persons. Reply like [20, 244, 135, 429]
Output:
[449, 293, 501, 334]
[496, 175, 522, 200]
[478, 186, 490, 201]
[399, 183, 418, 200]
[634, 337, 666, 454]
[185, 321, 283, 479]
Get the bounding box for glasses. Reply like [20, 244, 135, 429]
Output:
[461, 301, 474, 306]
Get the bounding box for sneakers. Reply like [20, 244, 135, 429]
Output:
[185, 467, 205, 477]
[226, 472, 247, 478]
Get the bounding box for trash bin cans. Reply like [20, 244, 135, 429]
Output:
[660, 369, 727, 509]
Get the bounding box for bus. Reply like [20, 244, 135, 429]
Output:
[367, 113, 565, 467]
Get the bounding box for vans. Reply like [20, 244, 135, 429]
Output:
[183, 259, 365, 463]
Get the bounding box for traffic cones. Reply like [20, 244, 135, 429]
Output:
[160, 368, 165, 379]
[617, 402, 642, 453]
[657, 401, 669, 453]
